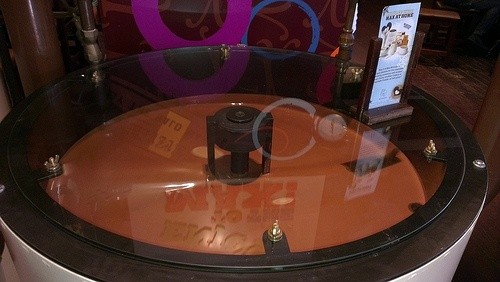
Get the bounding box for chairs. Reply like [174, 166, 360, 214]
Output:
[411, 0, 461, 65]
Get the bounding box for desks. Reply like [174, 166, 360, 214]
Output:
[1, 42, 488, 282]
